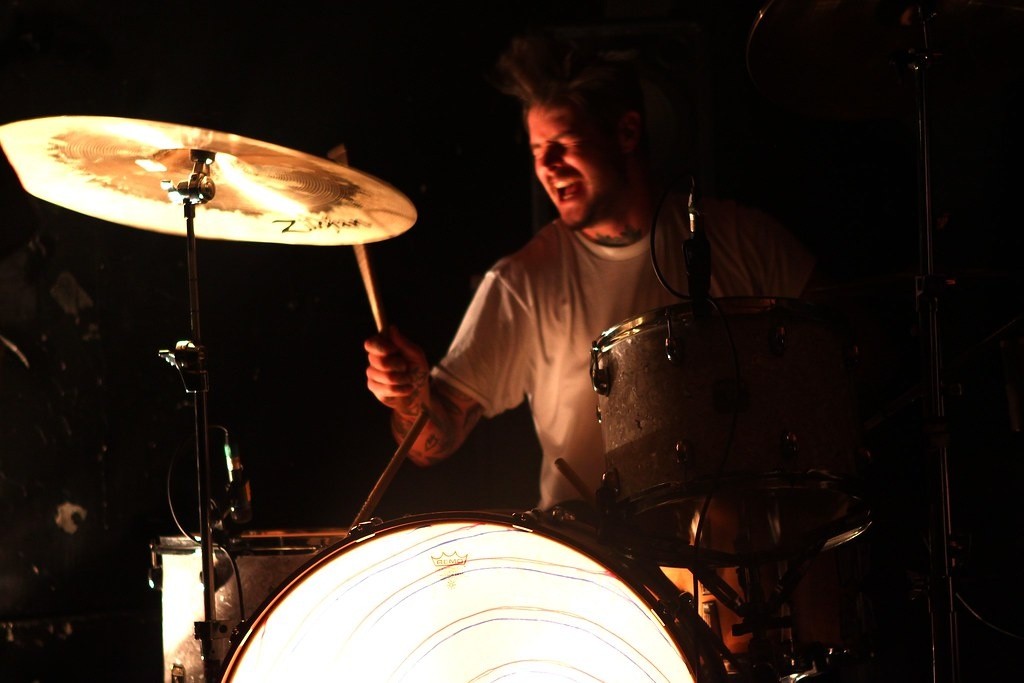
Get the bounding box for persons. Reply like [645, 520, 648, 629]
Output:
[360, 23, 848, 672]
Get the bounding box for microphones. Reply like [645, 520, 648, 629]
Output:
[221, 430, 254, 525]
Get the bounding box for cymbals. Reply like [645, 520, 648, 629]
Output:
[740, 0, 1004, 119]
[1, 113, 417, 247]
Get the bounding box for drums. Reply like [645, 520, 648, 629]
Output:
[588, 292, 879, 546]
[154, 502, 876, 683]
